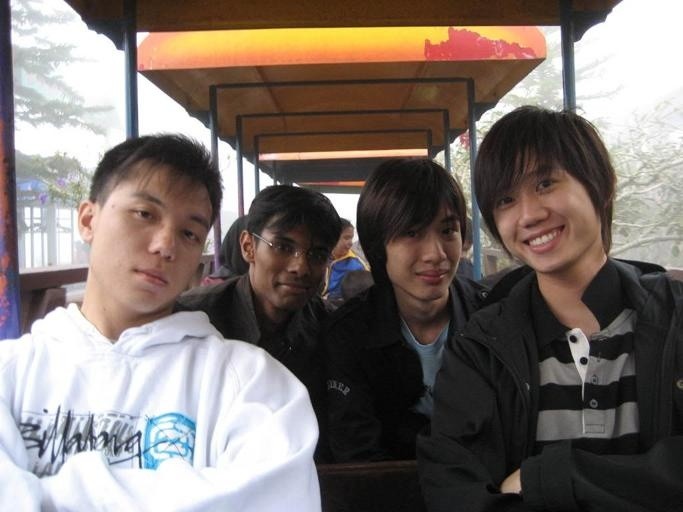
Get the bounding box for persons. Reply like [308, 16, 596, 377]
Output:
[199, 215, 248, 287]
[326, 270, 374, 310]
[321, 216, 370, 299]
[415, 105, 683, 511]
[325, 153, 493, 461]
[175, 185, 340, 463]
[455, 217, 485, 281]
[0, 131, 325, 512]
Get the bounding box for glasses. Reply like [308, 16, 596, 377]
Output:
[250, 231, 335, 268]
